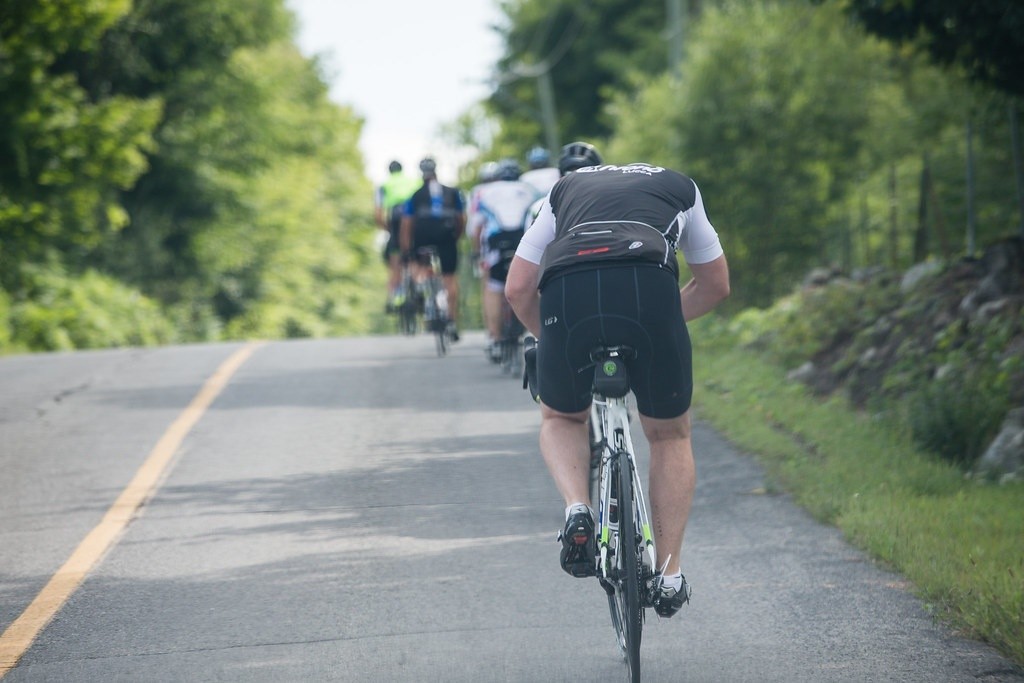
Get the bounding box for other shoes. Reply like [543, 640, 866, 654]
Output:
[386, 303, 393, 313]
[484, 341, 505, 366]
[448, 321, 460, 344]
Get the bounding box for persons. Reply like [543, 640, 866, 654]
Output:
[523, 142, 604, 234]
[503, 162, 730, 618]
[469, 147, 561, 362]
[379, 161, 425, 309]
[406, 157, 466, 342]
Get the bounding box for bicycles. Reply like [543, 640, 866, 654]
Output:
[375, 218, 421, 336]
[470, 248, 523, 380]
[521, 332, 662, 683]
[399, 243, 454, 358]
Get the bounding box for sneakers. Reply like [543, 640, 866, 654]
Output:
[560, 504, 596, 578]
[654, 573, 692, 618]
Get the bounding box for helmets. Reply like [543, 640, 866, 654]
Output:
[389, 160, 402, 172]
[558, 141, 602, 176]
[478, 162, 499, 181]
[494, 160, 520, 178]
[528, 147, 549, 164]
[420, 160, 436, 171]
